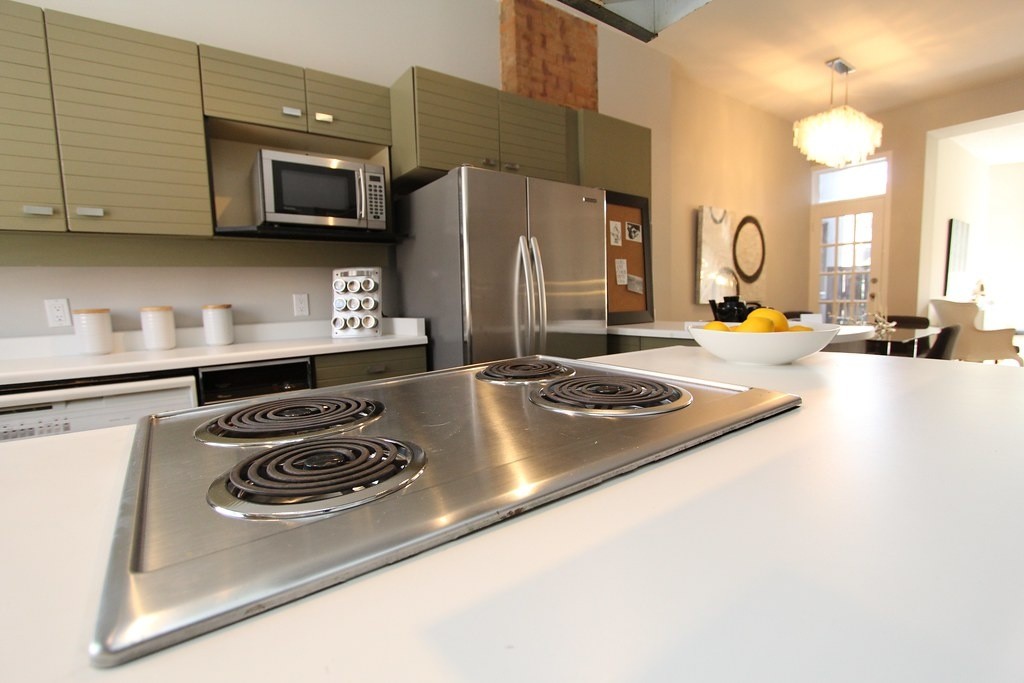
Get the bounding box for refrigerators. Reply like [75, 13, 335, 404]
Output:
[395, 166, 608, 373]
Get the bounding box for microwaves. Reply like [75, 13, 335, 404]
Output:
[249, 149, 387, 232]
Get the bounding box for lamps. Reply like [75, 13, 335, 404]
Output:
[791, 58, 886, 171]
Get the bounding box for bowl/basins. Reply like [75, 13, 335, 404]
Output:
[688, 322, 841, 366]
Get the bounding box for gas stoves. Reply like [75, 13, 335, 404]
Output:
[87, 354, 803, 669]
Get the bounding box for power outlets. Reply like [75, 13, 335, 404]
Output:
[43, 298, 72, 327]
[293, 293, 310, 317]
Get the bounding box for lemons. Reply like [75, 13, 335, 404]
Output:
[702, 308, 813, 333]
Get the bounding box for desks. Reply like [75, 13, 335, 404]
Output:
[864, 324, 942, 358]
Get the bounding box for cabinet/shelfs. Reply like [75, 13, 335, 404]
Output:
[315, 345, 435, 387]
[392, 66, 580, 184]
[0, 1, 213, 238]
[198, 40, 392, 150]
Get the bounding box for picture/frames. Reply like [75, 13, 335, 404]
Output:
[606, 188, 655, 327]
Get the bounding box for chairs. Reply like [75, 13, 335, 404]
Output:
[930, 322, 961, 360]
[868, 315, 931, 357]
